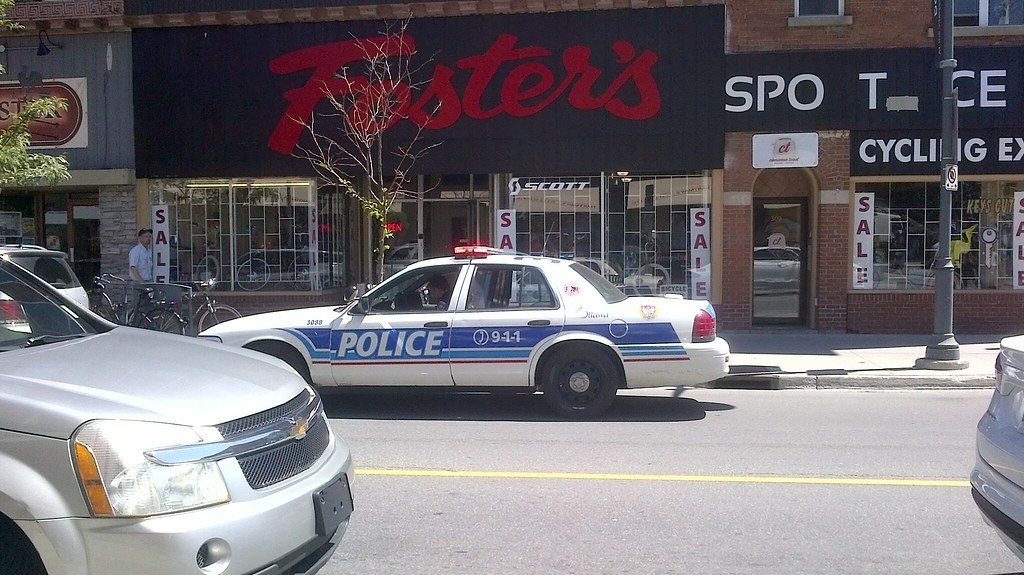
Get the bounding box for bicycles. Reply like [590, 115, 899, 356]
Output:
[85, 271, 242, 336]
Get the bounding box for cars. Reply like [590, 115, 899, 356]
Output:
[519, 255, 666, 302]
[969, 332, 1024, 564]
[197, 244, 730, 422]
[0, 243, 355, 575]
[688, 246, 801, 319]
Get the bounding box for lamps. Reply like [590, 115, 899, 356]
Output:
[37, 29, 64, 56]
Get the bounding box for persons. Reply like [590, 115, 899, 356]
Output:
[300, 241, 309, 279]
[129, 227, 154, 329]
[428, 276, 451, 310]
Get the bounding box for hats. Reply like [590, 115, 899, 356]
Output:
[426, 275, 448, 289]
[138, 228, 153, 237]
